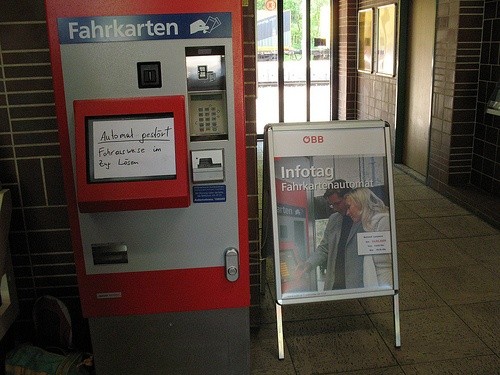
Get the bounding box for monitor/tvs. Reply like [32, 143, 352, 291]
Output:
[85, 112, 177, 184]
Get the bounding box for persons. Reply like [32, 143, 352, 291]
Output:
[296, 180, 393, 291]
